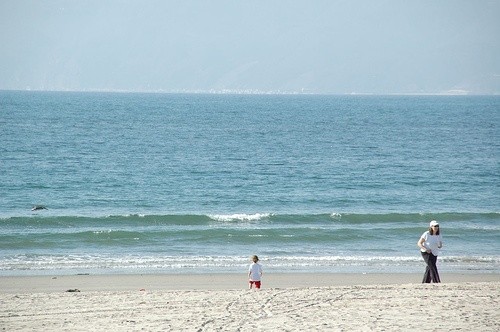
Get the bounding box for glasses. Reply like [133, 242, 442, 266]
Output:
[434, 225, 439, 227]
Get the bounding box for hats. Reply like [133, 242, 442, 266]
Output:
[429, 220, 439, 226]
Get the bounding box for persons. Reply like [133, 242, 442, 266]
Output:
[418, 220, 444, 284]
[245, 255, 264, 288]
